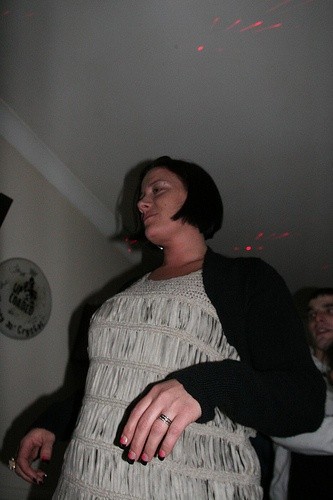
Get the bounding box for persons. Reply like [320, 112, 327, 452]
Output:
[269, 287, 333, 500]
[0, 155, 328, 500]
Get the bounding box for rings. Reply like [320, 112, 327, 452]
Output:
[159, 413, 172, 426]
[9, 457, 16, 473]
